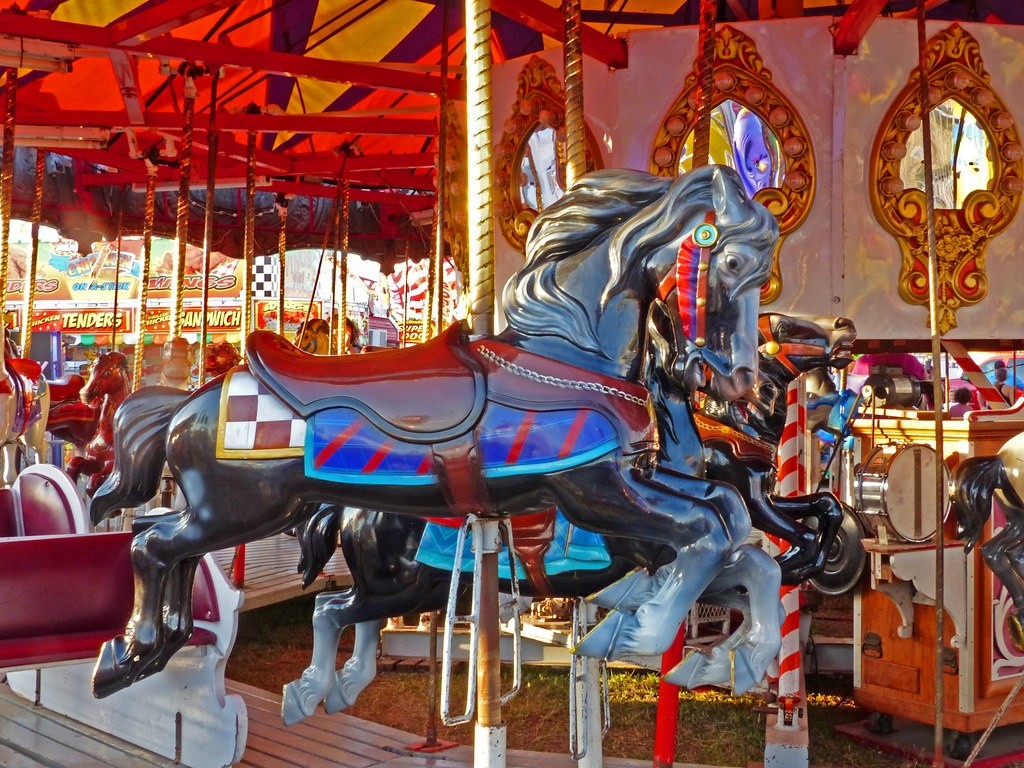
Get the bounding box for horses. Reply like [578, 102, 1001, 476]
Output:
[2, 162, 1023, 727]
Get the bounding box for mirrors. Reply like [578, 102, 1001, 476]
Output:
[872, 23, 1024, 336]
[647, 25, 816, 306]
[492, 54, 605, 259]
